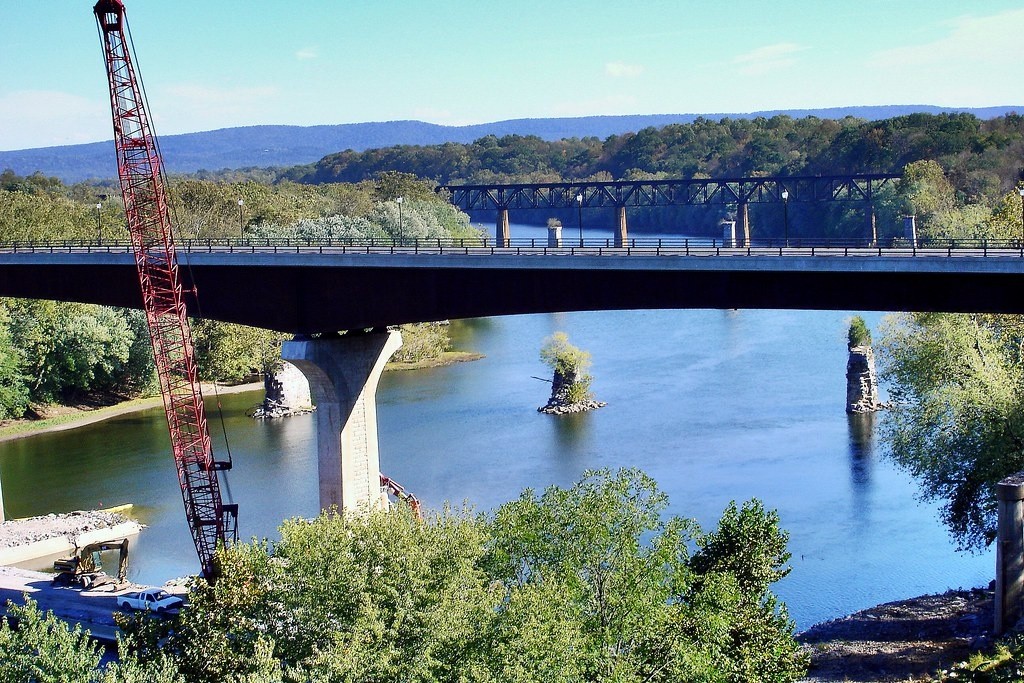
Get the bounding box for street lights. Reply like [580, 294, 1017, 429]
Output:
[397, 195, 403, 246]
[577, 194, 584, 247]
[1018, 189, 1024, 222]
[97, 202, 103, 246]
[238, 199, 244, 247]
[782, 189, 789, 247]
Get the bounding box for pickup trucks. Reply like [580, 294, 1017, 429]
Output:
[116, 588, 183, 614]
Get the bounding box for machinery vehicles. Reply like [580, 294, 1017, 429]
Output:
[380, 472, 420, 522]
[92, 0, 242, 582]
[54, 538, 132, 594]
